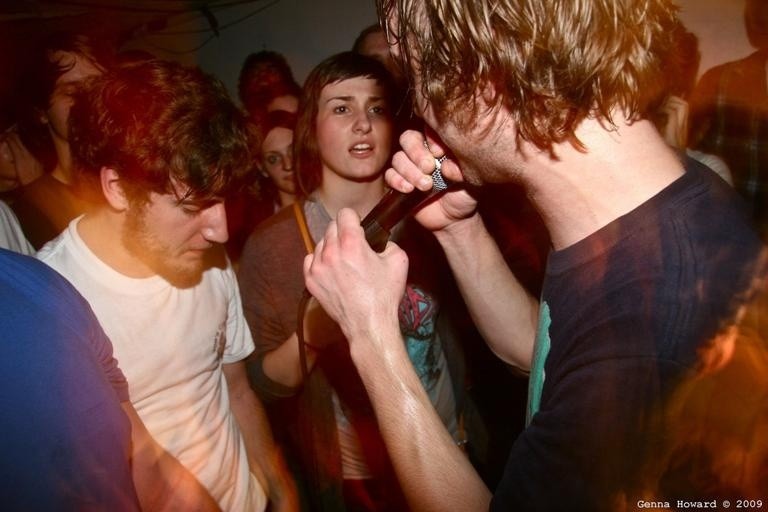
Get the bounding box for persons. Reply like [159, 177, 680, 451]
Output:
[0, 0, 767, 510]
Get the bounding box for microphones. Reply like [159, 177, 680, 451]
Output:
[360, 142, 452, 250]
